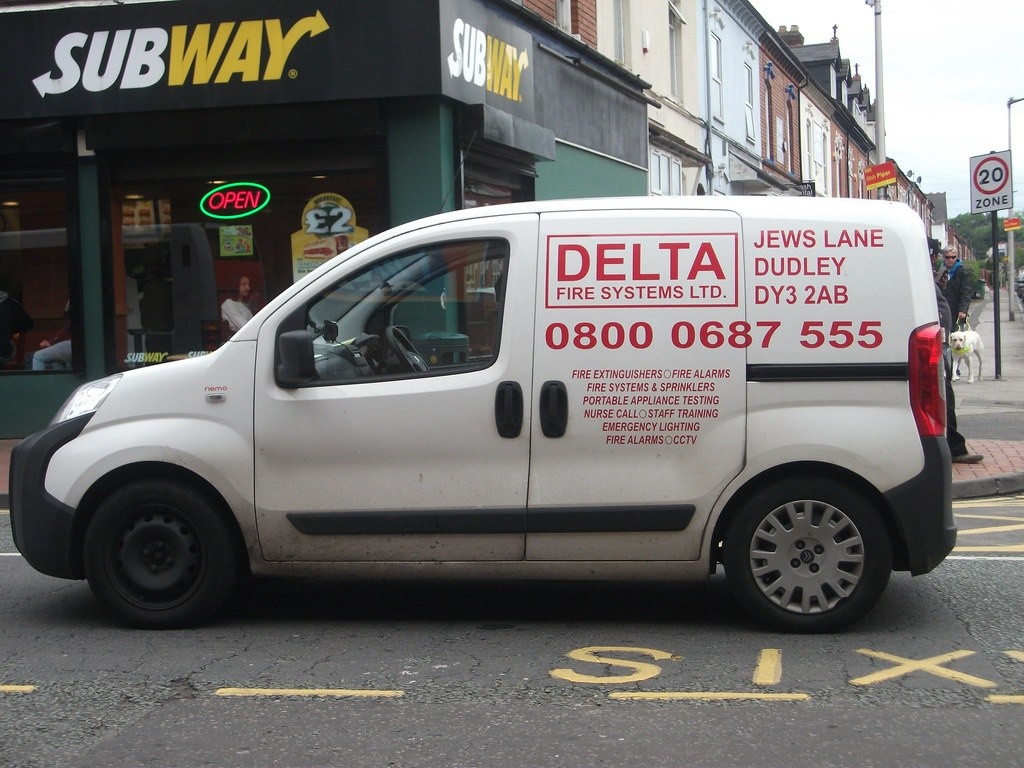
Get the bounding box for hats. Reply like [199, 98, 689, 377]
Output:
[928, 238, 938, 255]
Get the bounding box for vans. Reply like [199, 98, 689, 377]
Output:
[9, 196, 956, 631]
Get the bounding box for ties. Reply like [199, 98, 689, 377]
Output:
[940, 270, 948, 288]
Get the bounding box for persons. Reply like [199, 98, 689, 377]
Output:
[0, 276, 34, 370]
[219, 272, 253, 347]
[33, 326, 71, 371]
[927, 237, 983, 464]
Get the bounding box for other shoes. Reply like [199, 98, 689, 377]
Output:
[952, 454, 983, 463]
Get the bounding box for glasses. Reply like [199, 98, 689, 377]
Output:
[944, 254, 956, 259]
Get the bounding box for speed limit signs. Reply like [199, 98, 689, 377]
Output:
[973, 157, 1009, 195]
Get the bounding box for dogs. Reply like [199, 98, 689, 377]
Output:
[948, 331, 986, 383]
